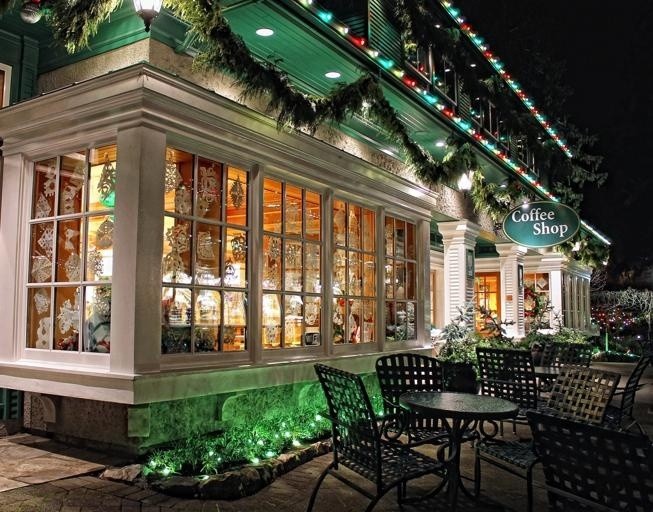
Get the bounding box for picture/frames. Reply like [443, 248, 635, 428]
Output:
[466, 248, 475, 280]
[517, 263, 523, 288]
[534, 271, 550, 293]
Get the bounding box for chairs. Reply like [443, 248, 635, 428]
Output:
[474, 365, 620, 509]
[525, 412, 652, 510]
[375, 352, 481, 499]
[309, 363, 453, 511]
[475, 342, 652, 436]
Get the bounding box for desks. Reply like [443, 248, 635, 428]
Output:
[399, 391, 520, 511]
